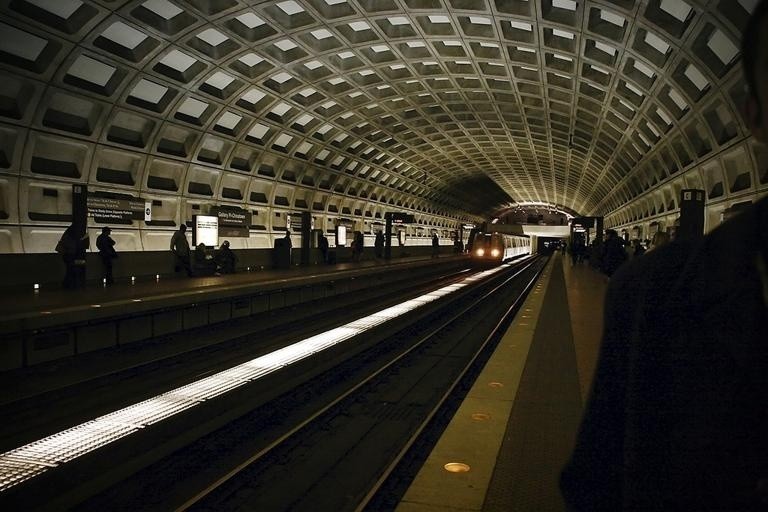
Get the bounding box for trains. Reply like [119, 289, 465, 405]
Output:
[469, 230, 532, 267]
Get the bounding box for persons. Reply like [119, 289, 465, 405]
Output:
[217, 241, 240, 275]
[350, 230, 386, 267]
[559, 0, 766, 511]
[431, 235, 439, 258]
[96, 227, 118, 288]
[196, 243, 221, 277]
[284, 231, 292, 269]
[559, 228, 678, 277]
[170, 224, 195, 279]
